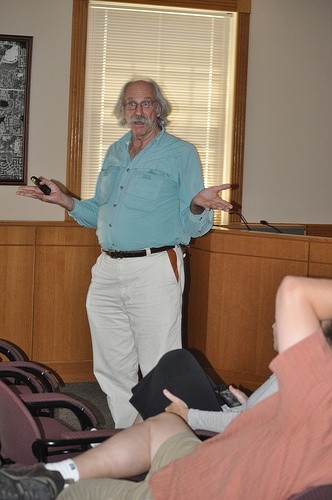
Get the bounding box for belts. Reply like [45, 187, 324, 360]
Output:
[102, 246, 175, 259]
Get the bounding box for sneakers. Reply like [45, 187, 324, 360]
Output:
[0, 463, 65, 500]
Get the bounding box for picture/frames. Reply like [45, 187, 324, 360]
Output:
[0, 34, 33, 186]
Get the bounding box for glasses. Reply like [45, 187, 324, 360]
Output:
[126, 100, 154, 110]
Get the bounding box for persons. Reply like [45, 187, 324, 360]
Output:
[15, 80, 235, 431]
[0, 276, 332, 500]
[130, 318, 332, 434]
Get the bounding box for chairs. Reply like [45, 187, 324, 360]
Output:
[0, 338, 128, 468]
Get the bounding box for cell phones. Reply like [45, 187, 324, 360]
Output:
[216, 384, 241, 407]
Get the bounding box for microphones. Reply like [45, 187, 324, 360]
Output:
[260, 221, 282, 232]
[229, 212, 250, 230]
